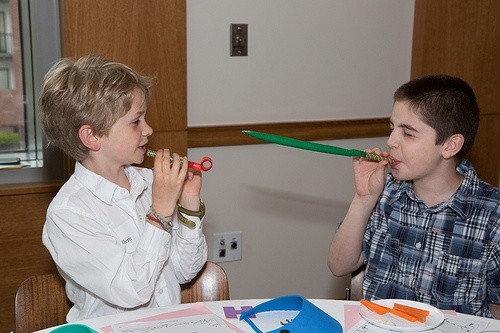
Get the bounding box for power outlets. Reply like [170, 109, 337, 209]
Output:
[212, 231, 242, 261]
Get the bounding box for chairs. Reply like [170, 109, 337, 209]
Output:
[15, 260, 229, 333]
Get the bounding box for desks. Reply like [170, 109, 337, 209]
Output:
[31, 298, 500, 333]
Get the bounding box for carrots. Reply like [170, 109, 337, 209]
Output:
[359, 298, 430, 323]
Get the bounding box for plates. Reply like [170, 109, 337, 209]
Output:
[359, 299, 445, 332]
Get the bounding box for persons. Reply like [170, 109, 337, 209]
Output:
[40, 56, 209, 323]
[328, 75, 500, 321]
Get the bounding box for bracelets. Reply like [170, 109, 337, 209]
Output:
[146, 206, 172, 234]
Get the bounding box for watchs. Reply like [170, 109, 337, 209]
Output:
[177, 200, 205, 227]
